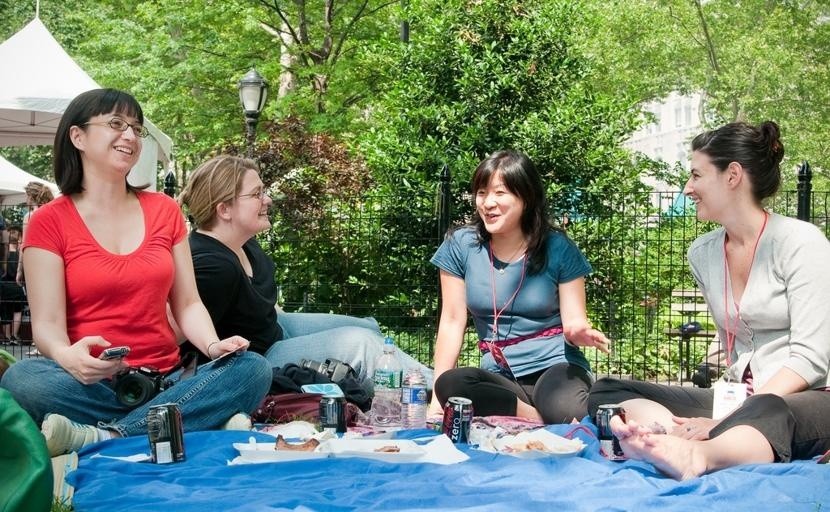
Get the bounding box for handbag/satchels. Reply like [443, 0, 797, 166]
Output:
[251, 393, 357, 427]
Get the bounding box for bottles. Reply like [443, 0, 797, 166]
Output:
[401, 363, 428, 431]
[370, 336, 403, 429]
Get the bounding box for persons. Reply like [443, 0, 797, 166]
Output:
[587, 120, 830, 484]
[424, 149, 612, 430]
[1, 88, 275, 457]
[0, 182, 55, 356]
[166, 154, 436, 394]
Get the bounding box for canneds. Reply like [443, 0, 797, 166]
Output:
[319, 396, 347, 432]
[146, 402, 186, 463]
[442, 395, 475, 443]
[595, 404, 628, 462]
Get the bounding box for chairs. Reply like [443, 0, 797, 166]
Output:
[665, 290, 717, 365]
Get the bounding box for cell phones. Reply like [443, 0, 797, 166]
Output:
[99, 346, 130, 361]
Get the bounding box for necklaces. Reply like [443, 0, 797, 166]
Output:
[493, 237, 525, 275]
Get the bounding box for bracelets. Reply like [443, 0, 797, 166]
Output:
[208, 341, 217, 361]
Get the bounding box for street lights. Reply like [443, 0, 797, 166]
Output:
[236, 66, 270, 156]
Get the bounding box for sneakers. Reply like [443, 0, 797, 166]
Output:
[41, 412, 98, 457]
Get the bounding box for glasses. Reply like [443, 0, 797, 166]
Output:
[84, 118, 151, 138]
[240, 188, 267, 198]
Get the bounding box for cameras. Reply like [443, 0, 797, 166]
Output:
[113, 368, 173, 406]
[300, 357, 357, 383]
